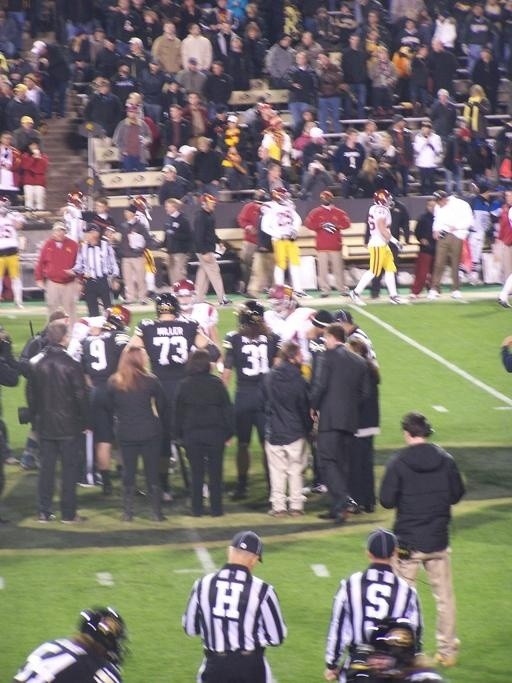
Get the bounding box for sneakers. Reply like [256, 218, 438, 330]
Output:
[295, 289, 312, 299]
[311, 485, 328, 494]
[427, 289, 443, 300]
[103, 481, 112, 495]
[60, 512, 88, 524]
[497, 292, 512, 308]
[388, 295, 407, 305]
[19, 453, 39, 472]
[450, 291, 469, 305]
[162, 490, 172, 502]
[142, 298, 155, 305]
[218, 298, 233, 306]
[268, 509, 288, 517]
[288, 509, 302, 516]
[37, 511, 57, 523]
[349, 290, 367, 307]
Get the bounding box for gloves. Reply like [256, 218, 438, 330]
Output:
[282, 234, 291, 241]
[290, 229, 297, 240]
[389, 235, 403, 253]
[321, 221, 339, 235]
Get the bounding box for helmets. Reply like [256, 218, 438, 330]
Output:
[67, 190, 83, 207]
[374, 188, 392, 206]
[267, 283, 298, 320]
[318, 190, 334, 204]
[174, 278, 195, 296]
[105, 304, 131, 324]
[0, 196, 11, 206]
[271, 186, 288, 198]
[79, 605, 127, 647]
[238, 300, 264, 323]
[372, 617, 417, 656]
[155, 293, 180, 318]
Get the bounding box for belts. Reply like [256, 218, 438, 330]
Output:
[207, 650, 255, 656]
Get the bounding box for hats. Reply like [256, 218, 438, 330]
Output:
[227, 116, 239, 124]
[49, 311, 69, 321]
[230, 531, 263, 563]
[13, 84, 28, 92]
[393, 113, 405, 122]
[128, 37, 144, 50]
[52, 221, 67, 230]
[126, 204, 137, 213]
[197, 192, 220, 203]
[20, 115, 34, 124]
[368, 528, 396, 557]
[83, 223, 101, 233]
[30, 40, 48, 56]
[150, 56, 160, 64]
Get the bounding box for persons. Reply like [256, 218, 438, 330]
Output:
[222, 302, 283, 500]
[189, 192, 232, 306]
[377, 412, 465, 668]
[256, 283, 317, 437]
[116, 293, 223, 471]
[302, 190, 352, 297]
[246, 218, 274, 299]
[33, 219, 84, 318]
[32, 40, 72, 118]
[462, 84, 492, 147]
[81, 198, 118, 242]
[396, 0, 511, 82]
[412, 119, 445, 194]
[258, 341, 314, 518]
[16, 310, 73, 469]
[500, 336, 512, 373]
[237, 186, 272, 295]
[266, 34, 298, 89]
[58, 191, 87, 243]
[0, 326, 19, 527]
[333, 309, 379, 370]
[283, 51, 319, 137]
[79, 0, 265, 118]
[130, 193, 161, 292]
[442, 127, 471, 197]
[156, 111, 384, 187]
[163, 197, 195, 287]
[408, 199, 439, 299]
[348, 189, 406, 307]
[174, 351, 236, 517]
[386, 114, 414, 197]
[316, 54, 345, 133]
[180, 531, 287, 683]
[105, 346, 167, 522]
[427, 89, 457, 138]
[308, 326, 370, 524]
[11, 605, 129, 683]
[368, 47, 396, 112]
[365, 196, 410, 300]
[268, 0, 396, 46]
[112, 205, 150, 305]
[0, 196, 27, 310]
[0, 0, 80, 60]
[341, 32, 371, 119]
[425, 190, 474, 304]
[345, 335, 381, 515]
[346, 621, 445, 683]
[321, 528, 423, 683]
[260, 186, 310, 298]
[496, 202, 512, 308]
[0, 131, 21, 198]
[19, 137, 48, 210]
[0, 50, 50, 155]
[497, 185, 512, 283]
[74, 306, 131, 498]
[25, 322, 95, 525]
[62, 223, 120, 316]
[110, 92, 153, 172]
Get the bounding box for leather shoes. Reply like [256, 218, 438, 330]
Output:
[318, 512, 336, 520]
[334, 498, 358, 525]
